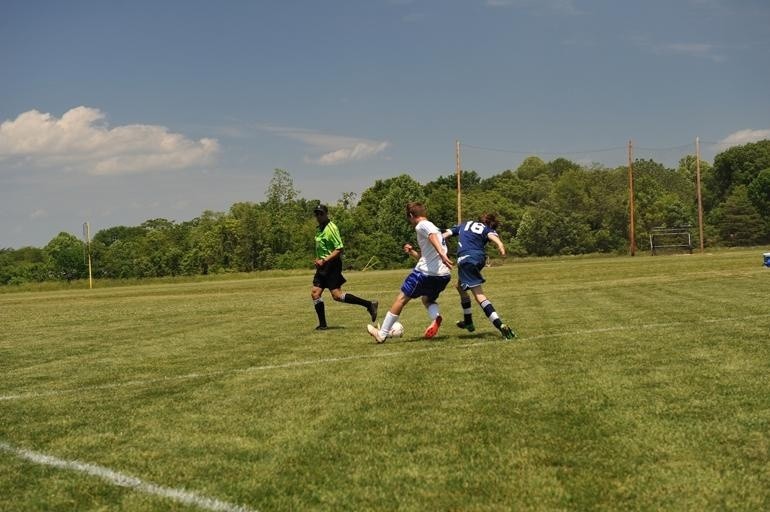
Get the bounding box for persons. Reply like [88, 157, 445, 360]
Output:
[367, 201, 454, 344]
[311, 204, 378, 330]
[442, 211, 518, 338]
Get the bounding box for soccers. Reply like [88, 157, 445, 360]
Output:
[387, 322, 405, 338]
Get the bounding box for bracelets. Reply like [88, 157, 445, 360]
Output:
[322, 259, 325, 263]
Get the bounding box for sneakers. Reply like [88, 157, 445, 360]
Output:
[424, 316, 442, 337]
[316, 326, 328, 330]
[367, 324, 386, 344]
[457, 321, 474, 332]
[501, 324, 517, 339]
[367, 302, 378, 322]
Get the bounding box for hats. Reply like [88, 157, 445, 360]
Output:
[313, 205, 328, 213]
